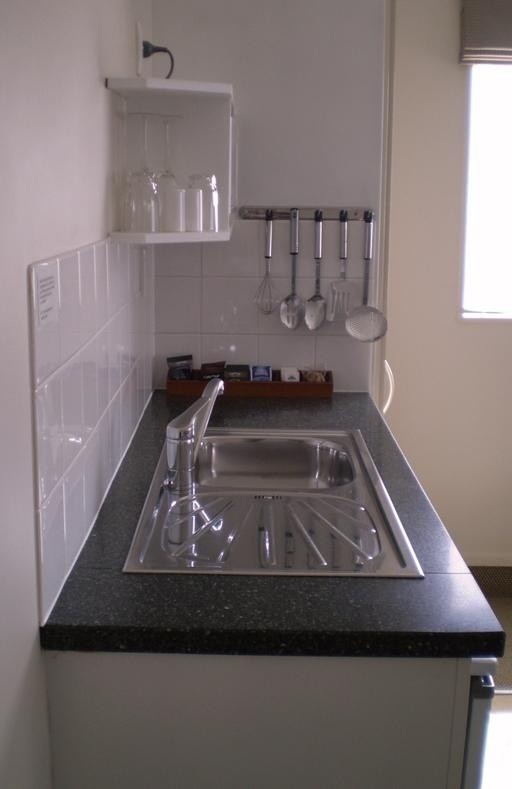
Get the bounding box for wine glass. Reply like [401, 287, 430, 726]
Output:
[153, 113, 178, 234]
[124, 108, 162, 232]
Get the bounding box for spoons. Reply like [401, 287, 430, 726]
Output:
[346, 210, 386, 343]
[305, 211, 327, 332]
[279, 210, 306, 331]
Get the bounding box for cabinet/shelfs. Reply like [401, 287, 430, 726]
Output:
[42, 390, 504, 786]
[108, 77, 240, 244]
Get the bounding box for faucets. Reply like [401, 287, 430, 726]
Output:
[166, 377, 223, 491]
[168, 493, 223, 564]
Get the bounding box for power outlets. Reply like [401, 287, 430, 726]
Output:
[135, 19, 156, 76]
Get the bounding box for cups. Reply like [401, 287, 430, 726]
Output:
[184, 188, 205, 232]
[166, 188, 186, 233]
[189, 166, 219, 233]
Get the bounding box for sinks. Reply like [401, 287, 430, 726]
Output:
[162, 432, 368, 496]
